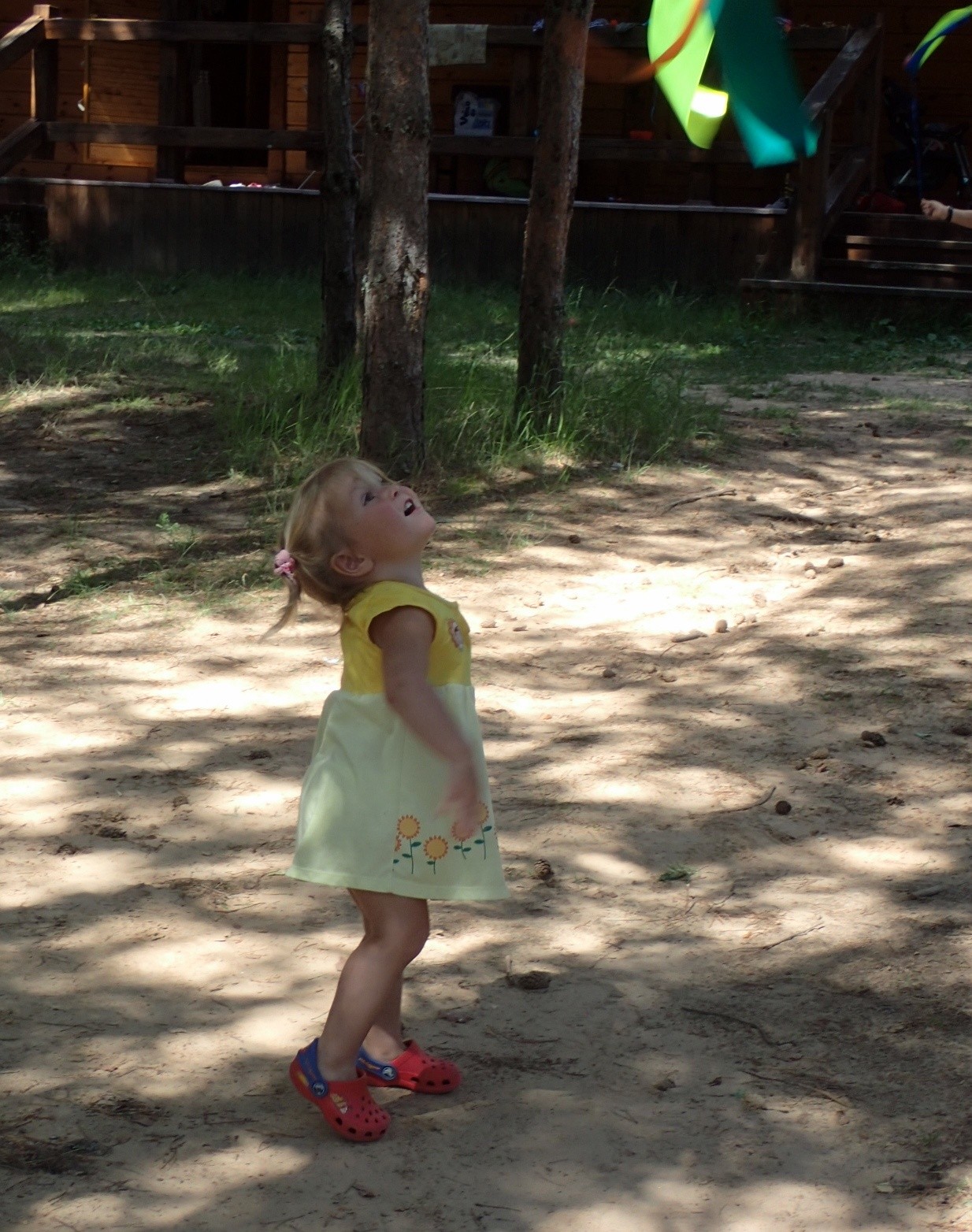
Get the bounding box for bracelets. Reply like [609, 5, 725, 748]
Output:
[945, 207, 953, 223]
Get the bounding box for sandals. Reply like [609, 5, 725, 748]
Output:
[358, 1036, 460, 1097]
[289, 1035, 389, 1142]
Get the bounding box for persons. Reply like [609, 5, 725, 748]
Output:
[260, 456, 506, 1143]
[920, 199, 972, 228]
